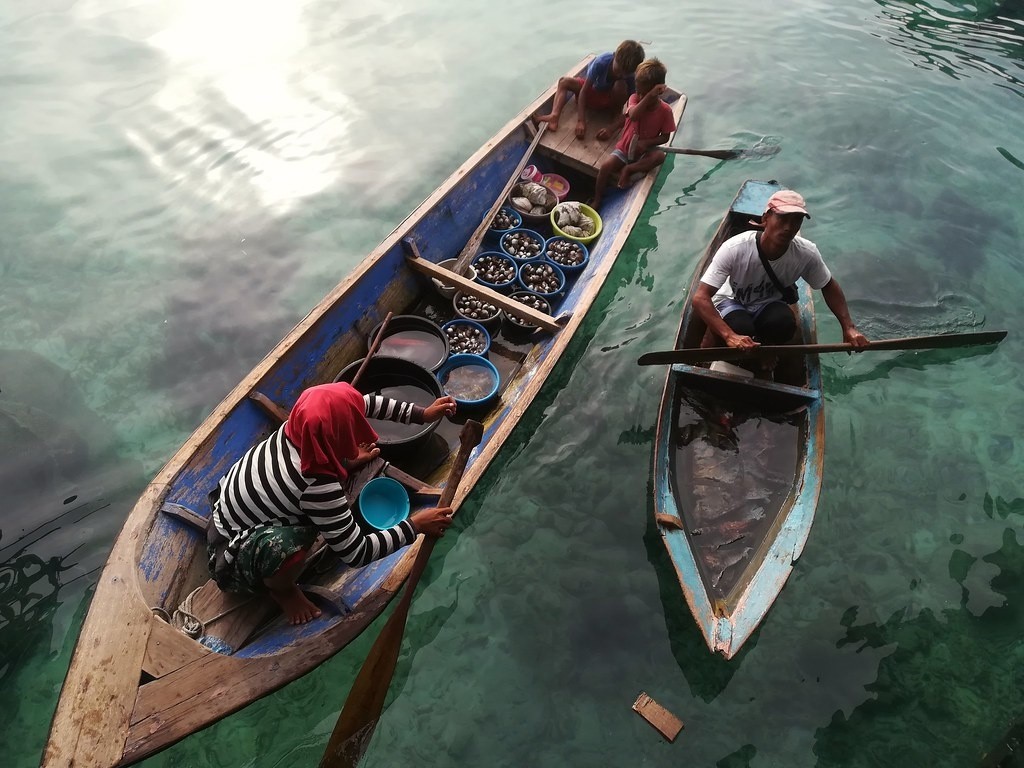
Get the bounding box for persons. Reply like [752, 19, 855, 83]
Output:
[691, 190, 871, 382]
[207, 382, 456, 625]
[533, 40, 645, 141]
[590, 56, 677, 211]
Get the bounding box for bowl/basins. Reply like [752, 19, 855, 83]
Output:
[543, 173, 570, 201]
[332, 354, 445, 453]
[367, 315, 450, 374]
[503, 291, 552, 329]
[508, 181, 559, 225]
[437, 354, 500, 407]
[483, 206, 523, 239]
[471, 251, 519, 290]
[545, 235, 589, 270]
[518, 260, 566, 300]
[453, 289, 502, 322]
[499, 228, 546, 265]
[441, 318, 491, 356]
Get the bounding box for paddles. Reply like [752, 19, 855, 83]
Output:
[628, 133, 782, 161]
[318, 418, 484, 768]
[636, 329, 1008, 366]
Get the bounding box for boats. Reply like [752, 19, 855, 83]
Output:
[38, 51, 688, 768]
[651, 176, 824, 662]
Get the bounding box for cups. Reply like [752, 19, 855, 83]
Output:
[521, 164, 543, 184]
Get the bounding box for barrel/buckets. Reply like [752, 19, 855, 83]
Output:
[550, 202, 603, 245]
[358, 477, 411, 530]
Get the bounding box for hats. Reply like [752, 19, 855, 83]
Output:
[763, 190, 811, 219]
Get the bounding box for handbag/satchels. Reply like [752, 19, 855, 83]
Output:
[782, 281, 799, 305]
